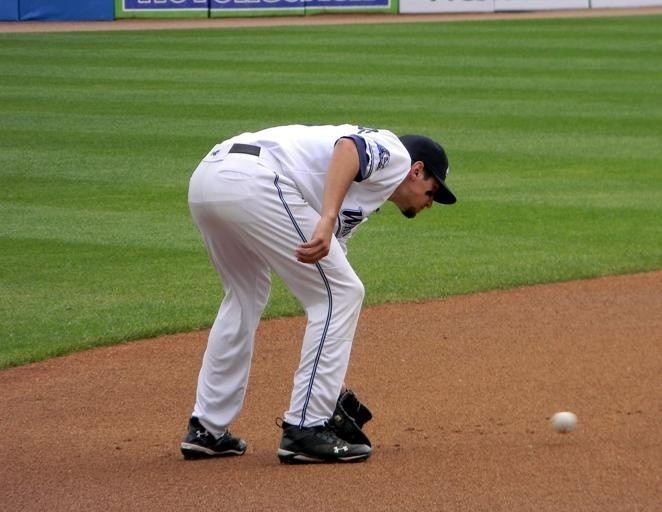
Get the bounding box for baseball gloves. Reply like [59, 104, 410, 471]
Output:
[325, 390, 371, 446]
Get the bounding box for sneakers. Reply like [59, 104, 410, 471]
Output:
[180, 416, 246, 458]
[277, 420, 372, 465]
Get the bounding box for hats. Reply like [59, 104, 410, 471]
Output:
[398, 134, 457, 204]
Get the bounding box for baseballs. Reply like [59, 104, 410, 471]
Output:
[551, 412, 576, 432]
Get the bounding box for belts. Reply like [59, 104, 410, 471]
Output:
[213, 144, 261, 156]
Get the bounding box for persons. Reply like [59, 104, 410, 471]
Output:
[179, 122, 458, 464]
